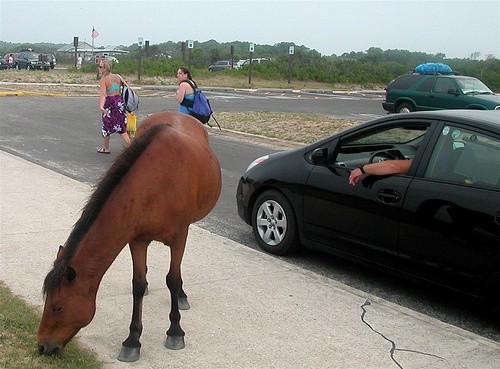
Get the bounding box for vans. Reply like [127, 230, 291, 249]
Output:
[239, 59, 269, 68]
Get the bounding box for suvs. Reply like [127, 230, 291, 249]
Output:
[381, 62, 500, 114]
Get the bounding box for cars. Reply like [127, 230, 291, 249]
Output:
[0, 47, 56, 71]
[154, 52, 172, 60]
[87, 55, 119, 65]
[234, 108, 500, 333]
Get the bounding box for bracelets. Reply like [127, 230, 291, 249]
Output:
[359, 165, 365, 173]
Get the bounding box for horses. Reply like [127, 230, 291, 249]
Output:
[38, 109, 223, 363]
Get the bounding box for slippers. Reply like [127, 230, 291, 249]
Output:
[98, 148, 110, 154]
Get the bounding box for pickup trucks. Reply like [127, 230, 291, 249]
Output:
[208, 60, 238, 73]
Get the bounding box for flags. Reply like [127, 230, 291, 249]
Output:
[91, 29, 99, 38]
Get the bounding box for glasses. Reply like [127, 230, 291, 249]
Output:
[99, 65, 105, 68]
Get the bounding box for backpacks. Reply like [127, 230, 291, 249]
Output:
[179, 79, 212, 124]
[117, 74, 139, 111]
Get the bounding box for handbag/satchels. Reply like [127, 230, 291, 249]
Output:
[127, 113, 137, 138]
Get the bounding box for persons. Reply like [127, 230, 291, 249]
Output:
[348, 159, 413, 186]
[175, 67, 195, 115]
[9, 54, 14, 65]
[76, 54, 82, 69]
[96, 61, 132, 153]
